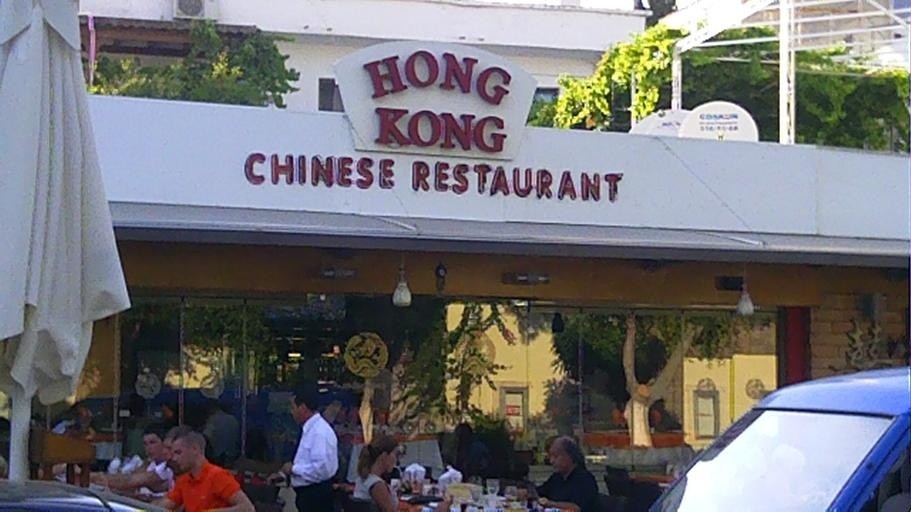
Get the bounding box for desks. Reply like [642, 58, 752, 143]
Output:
[631, 473, 678, 489]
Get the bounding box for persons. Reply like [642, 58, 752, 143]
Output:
[351, 433, 423, 512]
[266, 387, 342, 512]
[517, 434, 600, 512]
[0, 386, 269, 511]
[605, 396, 682, 434]
[449, 423, 500, 484]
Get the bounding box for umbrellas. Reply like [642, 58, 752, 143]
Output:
[0, 1, 138, 480]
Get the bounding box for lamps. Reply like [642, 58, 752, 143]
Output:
[734, 262, 757, 319]
[392, 246, 414, 305]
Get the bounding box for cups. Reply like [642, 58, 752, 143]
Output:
[409, 474, 541, 512]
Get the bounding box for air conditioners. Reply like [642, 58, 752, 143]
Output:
[172, 0, 220, 22]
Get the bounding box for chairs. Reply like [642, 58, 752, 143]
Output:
[36, 430, 97, 490]
[603, 464, 659, 509]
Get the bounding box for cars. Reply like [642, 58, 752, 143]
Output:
[0, 478, 173, 512]
[642, 367, 911, 510]
